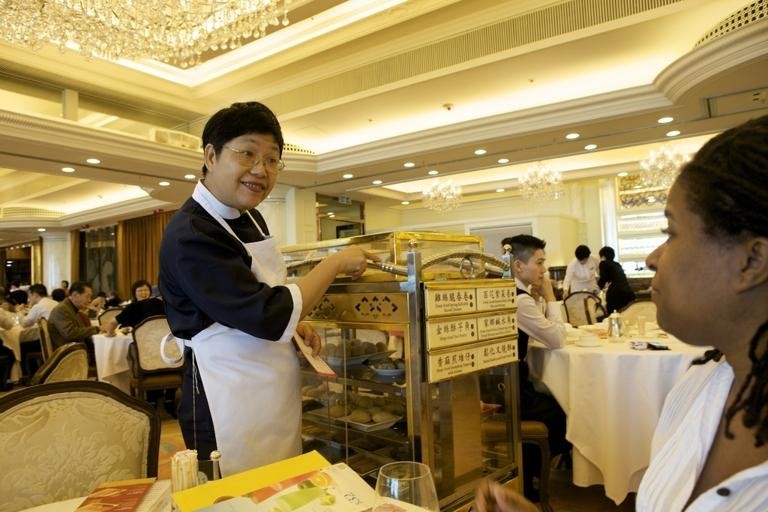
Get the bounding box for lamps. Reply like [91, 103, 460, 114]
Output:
[0, 0, 289, 69]
[421, 179, 464, 213]
[617, 138, 698, 210]
[516, 161, 565, 201]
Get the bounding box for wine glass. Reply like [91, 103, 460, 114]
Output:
[372, 461, 440, 512]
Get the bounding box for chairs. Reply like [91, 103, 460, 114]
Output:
[0, 379, 161, 511]
[564, 291, 601, 328]
[441, 416, 553, 512]
[98, 308, 124, 326]
[36, 317, 52, 362]
[618, 298, 658, 326]
[127, 315, 185, 418]
[29, 341, 88, 385]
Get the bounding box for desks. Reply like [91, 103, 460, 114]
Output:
[92, 332, 133, 387]
[528, 332, 711, 512]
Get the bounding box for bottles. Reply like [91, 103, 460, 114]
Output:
[607, 310, 625, 342]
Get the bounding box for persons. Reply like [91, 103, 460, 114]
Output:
[156, 100, 383, 480]
[593, 245, 635, 322]
[634, 114, 768, 512]
[0, 279, 179, 420]
[560, 244, 600, 300]
[507, 234, 566, 506]
[501, 238, 513, 278]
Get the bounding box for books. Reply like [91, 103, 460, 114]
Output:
[74, 450, 378, 512]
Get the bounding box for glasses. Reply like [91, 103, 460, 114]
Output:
[222, 144, 287, 175]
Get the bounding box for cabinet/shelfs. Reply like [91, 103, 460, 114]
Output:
[287, 237, 521, 511]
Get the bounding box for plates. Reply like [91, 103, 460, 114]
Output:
[307, 406, 404, 433]
[319, 350, 397, 367]
[564, 318, 661, 347]
[370, 363, 405, 376]
